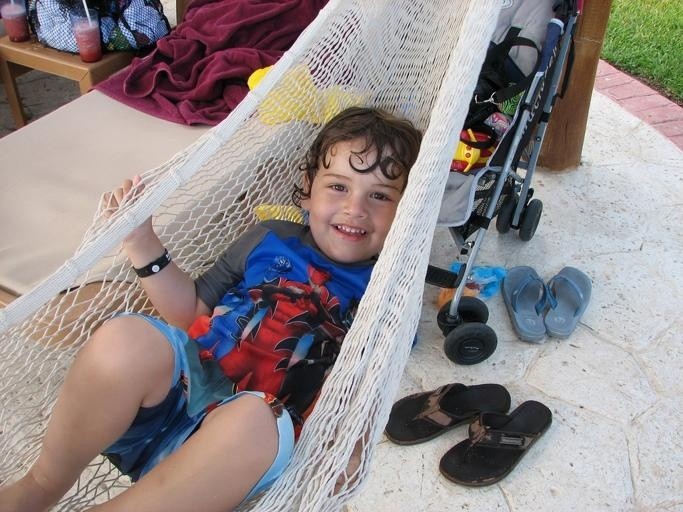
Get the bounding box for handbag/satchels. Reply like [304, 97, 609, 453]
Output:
[28, 0, 166, 52]
[464, 41, 530, 130]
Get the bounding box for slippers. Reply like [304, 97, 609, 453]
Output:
[501, 266, 547, 344]
[384, 383, 511, 445]
[439, 400, 555, 488]
[545, 267, 593, 338]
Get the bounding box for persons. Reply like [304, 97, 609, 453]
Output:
[1, 108, 440, 511]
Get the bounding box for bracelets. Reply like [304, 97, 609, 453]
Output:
[131, 249, 172, 276]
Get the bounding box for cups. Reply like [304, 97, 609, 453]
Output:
[0, 0, 31, 43]
[69, 9, 102, 63]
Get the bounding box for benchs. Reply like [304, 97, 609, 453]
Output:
[1, 47, 303, 348]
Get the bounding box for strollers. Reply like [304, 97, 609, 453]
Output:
[420, 1, 586, 364]
